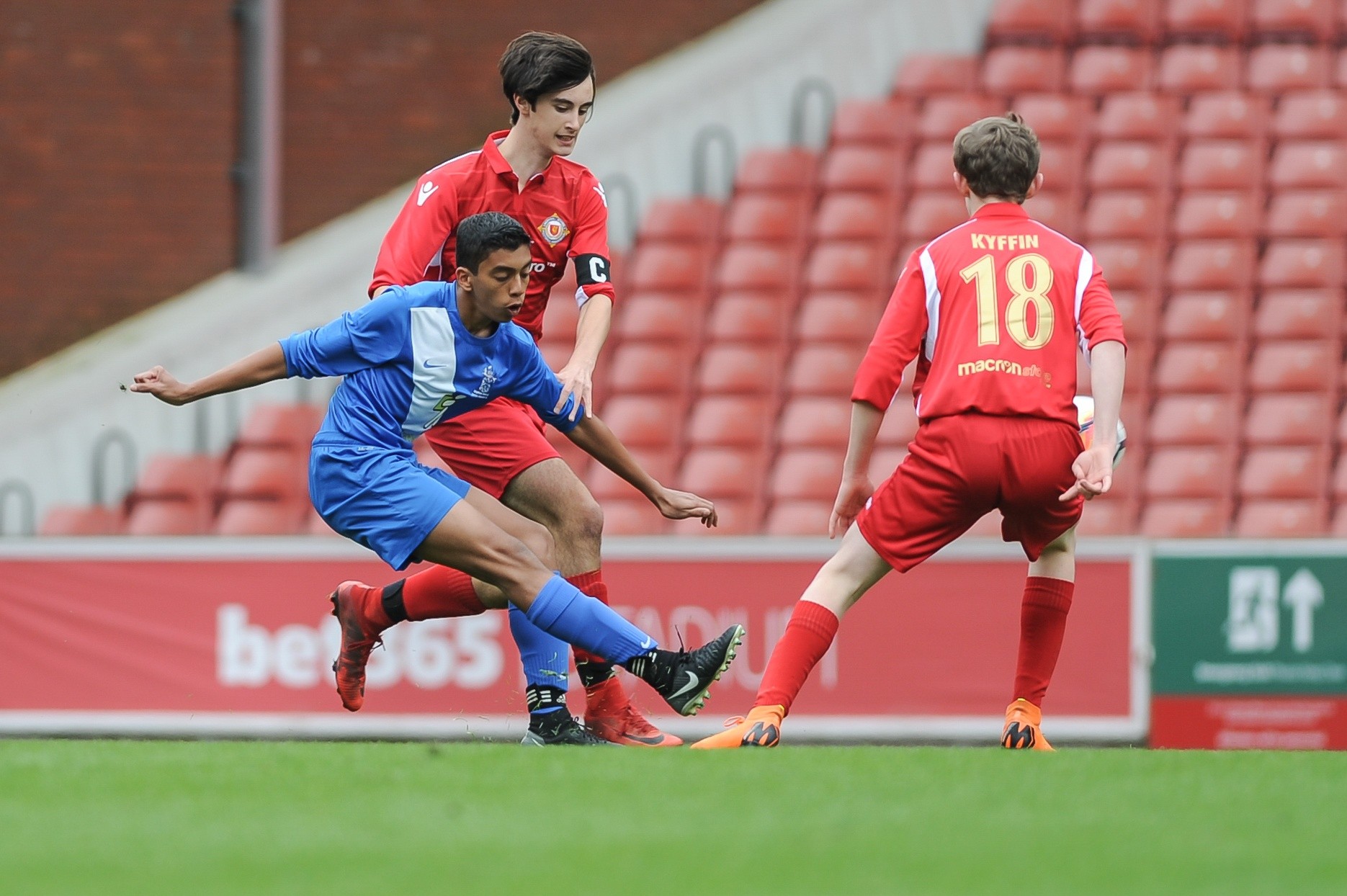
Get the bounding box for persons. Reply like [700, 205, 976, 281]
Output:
[332, 31, 683, 747]
[129, 210, 745, 746]
[693, 116, 1129, 750]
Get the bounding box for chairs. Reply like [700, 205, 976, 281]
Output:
[48, 1, 1347, 542]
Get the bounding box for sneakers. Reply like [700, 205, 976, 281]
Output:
[330, 581, 385, 712]
[689, 703, 785, 749]
[1000, 698, 1055, 750]
[520, 707, 625, 747]
[663, 624, 746, 717]
[584, 690, 684, 748]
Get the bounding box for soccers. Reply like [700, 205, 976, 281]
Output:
[1072, 395, 1127, 472]
